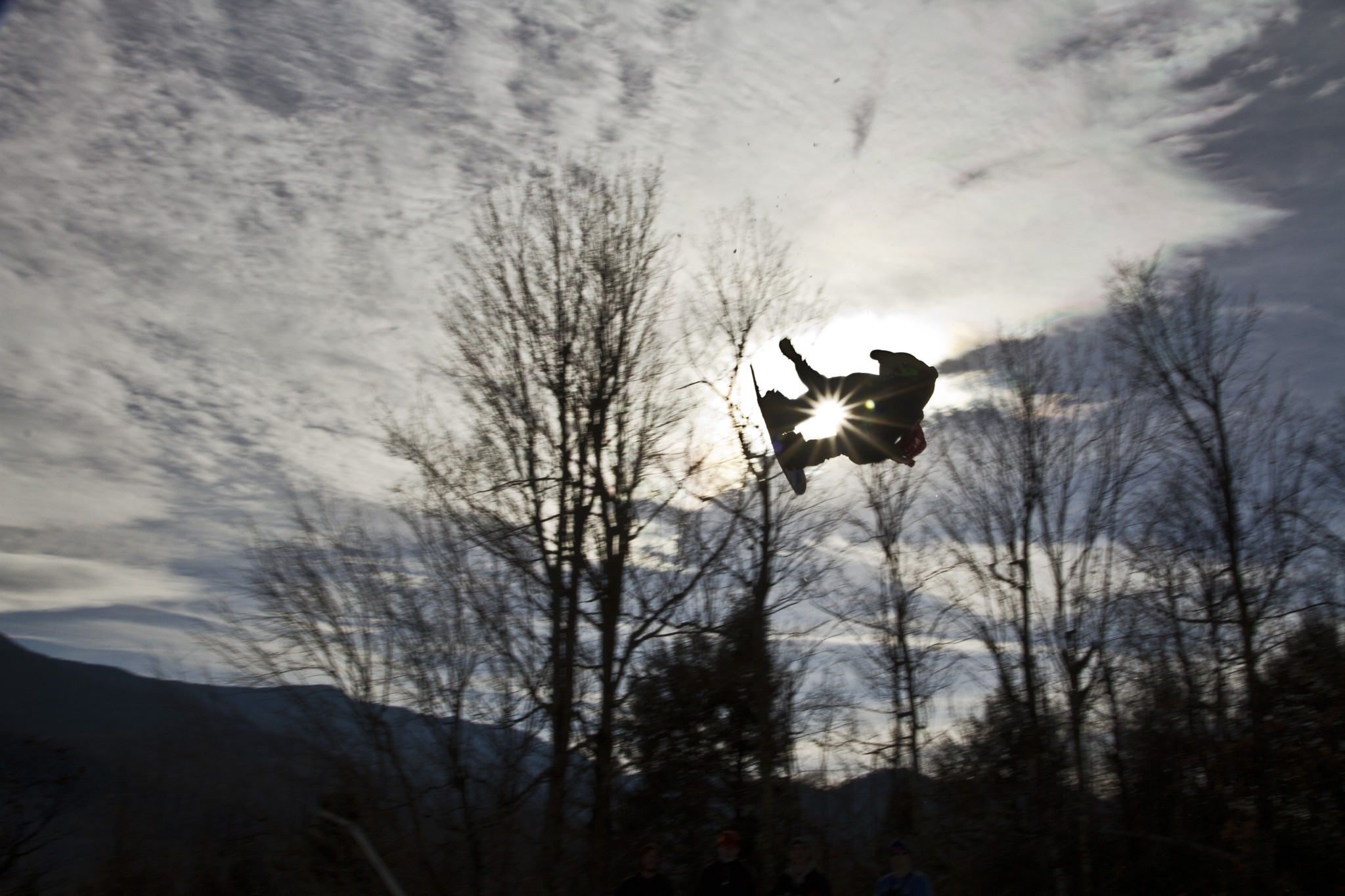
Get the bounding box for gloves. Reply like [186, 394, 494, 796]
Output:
[779, 338, 802, 363]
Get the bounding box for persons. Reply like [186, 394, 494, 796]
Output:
[760, 337, 938, 471]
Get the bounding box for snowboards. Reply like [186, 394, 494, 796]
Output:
[750, 363, 808, 495]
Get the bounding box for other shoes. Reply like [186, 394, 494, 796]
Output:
[767, 389, 795, 433]
[784, 432, 804, 471]
